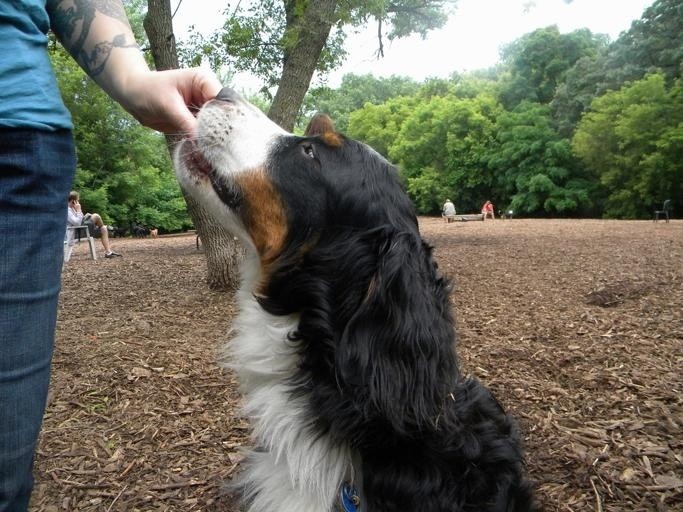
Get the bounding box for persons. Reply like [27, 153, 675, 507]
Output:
[67, 190, 123, 258]
[0, 1, 224, 512]
[305, 113, 335, 136]
[481, 200, 495, 221]
[442, 198, 457, 215]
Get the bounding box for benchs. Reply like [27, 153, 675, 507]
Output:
[446, 214, 483, 223]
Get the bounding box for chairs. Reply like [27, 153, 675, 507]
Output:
[65, 226, 97, 262]
[653, 200, 671, 224]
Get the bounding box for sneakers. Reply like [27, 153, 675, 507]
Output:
[107, 225, 118, 231]
[105, 252, 123, 258]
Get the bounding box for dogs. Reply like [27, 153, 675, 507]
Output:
[163, 86, 547, 512]
